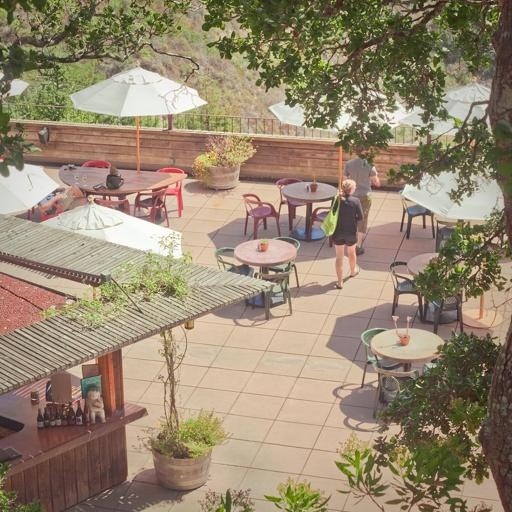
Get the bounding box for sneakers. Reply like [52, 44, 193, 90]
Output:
[357, 246, 364, 255]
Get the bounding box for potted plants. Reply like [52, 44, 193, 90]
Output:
[190, 131, 261, 191]
[133, 323, 235, 494]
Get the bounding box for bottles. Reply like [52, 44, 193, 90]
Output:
[36, 399, 84, 430]
[31, 391, 40, 403]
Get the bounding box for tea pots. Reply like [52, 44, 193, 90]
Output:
[104, 174, 124, 189]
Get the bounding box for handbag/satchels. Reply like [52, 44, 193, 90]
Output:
[320, 195, 341, 237]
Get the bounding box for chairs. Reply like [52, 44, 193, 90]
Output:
[214, 178, 341, 320]
[362, 325, 449, 405]
[390, 193, 484, 340]
[58, 159, 188, 228]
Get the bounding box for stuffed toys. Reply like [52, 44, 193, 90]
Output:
[85, 385, 105, 424]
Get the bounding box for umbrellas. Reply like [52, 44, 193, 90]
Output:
[402, 155, 505, 234]
[268, 80, 406, 196]
[69, 61, 207, 172]
[39, 195, 182, 262]
[398, 75, 493, 144]
[0, 158, 60, 217]
[0, 73, 30, 99]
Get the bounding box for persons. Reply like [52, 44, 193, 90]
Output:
[330, 179, 364, 288]
[344, 146, 380, 255]
